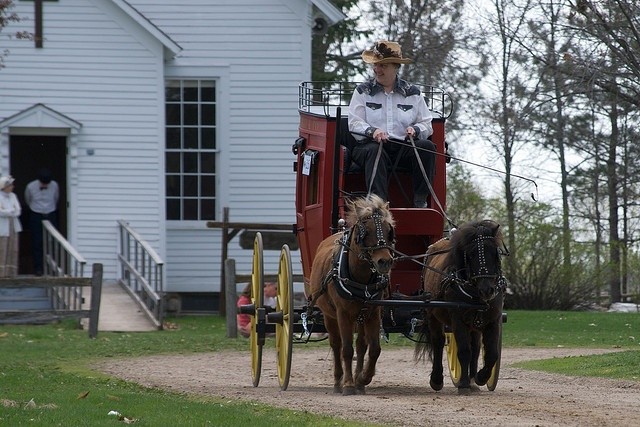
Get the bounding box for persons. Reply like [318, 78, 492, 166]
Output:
[0, 175, 24, 278]
[347, 41, 438, 209]
[25, 166, 60, 277]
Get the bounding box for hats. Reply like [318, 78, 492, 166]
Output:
[361, 42, 412, 64]
[38, 168, 51, 183]
[0, 175, 16, 191]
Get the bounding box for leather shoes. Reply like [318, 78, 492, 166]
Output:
[413, 192, 428, 208]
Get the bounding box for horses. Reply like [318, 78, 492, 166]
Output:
[308, 193, 397, 395]
[411, 217, 506, 394]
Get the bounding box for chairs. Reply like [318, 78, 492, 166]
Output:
[339, 145, 400, 174]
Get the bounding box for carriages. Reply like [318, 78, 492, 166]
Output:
[241, 81, 507, 396]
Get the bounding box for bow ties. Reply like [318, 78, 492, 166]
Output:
[38, 186, 47, 191]
